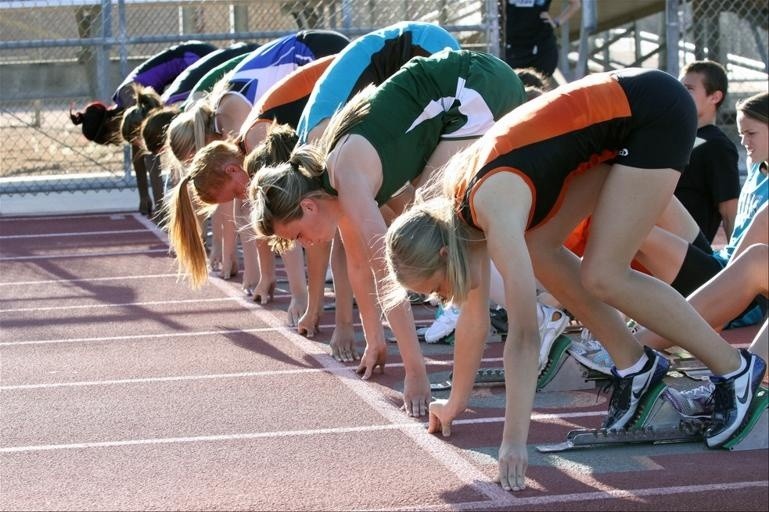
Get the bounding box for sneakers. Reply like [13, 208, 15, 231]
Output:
[705, 349, 767, 447]
[426, 300, 460, 342]
[535, 302, 669, 430]
[489, 308, 509, 333]
[667, 386, 715, 419]
